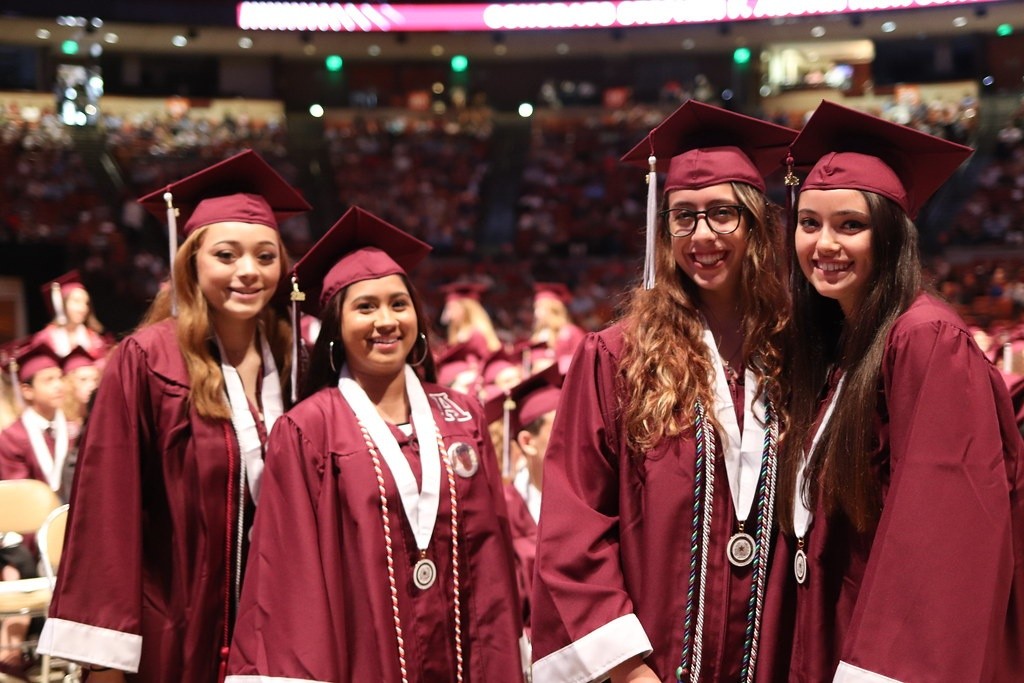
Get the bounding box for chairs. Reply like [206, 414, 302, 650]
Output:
[37, 501, 83, 683]
[0, 479, 60, 683]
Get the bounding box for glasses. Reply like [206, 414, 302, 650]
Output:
[660, 204, 748, 237]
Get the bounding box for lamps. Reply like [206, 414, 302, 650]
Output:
[492, 31, 504, 46]
[718, 24, 733, 36]
[394, 31, 410, 45]
[612, 28, 623, 42]
[850, 16, 864, 29]
[82, 22, 99, 36]
[973, 5, 988, 18]
[300, 30, 315, 44]
[187, 29, 202, 41]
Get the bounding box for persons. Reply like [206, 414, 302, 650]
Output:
[774, 100, 1024, 683]
[529, 97, 794, 683]
[223, 204, 526, 682]
[35, 148, 310, 683]
[0, 99, 1024, 683]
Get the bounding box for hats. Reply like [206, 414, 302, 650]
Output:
[484, 362, 564, 479]
[7, 271, 573, 414]
[787, 99, 975, 221]
[138, 149, 314, 319]
[619, 100, 800, 292]
[284, 207, 434, 404]
